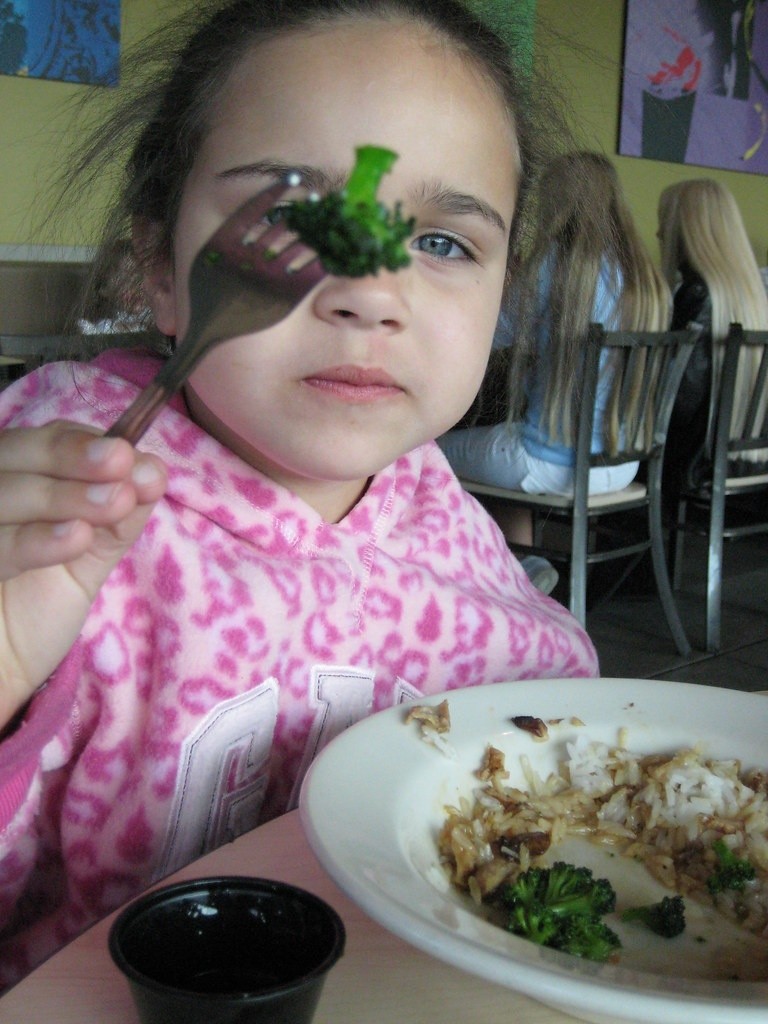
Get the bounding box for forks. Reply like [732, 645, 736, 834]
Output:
[102, 169, 331, 449]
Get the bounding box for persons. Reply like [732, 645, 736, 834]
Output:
[435, 151, 674, 593]
[601, 177, 768, 582]
[0, 0, 601, 996]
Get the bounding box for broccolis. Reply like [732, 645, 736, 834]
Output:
[262, 144, 415, 280]
[485, 836, 761, 963]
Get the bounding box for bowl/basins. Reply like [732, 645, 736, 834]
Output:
[108, 876, 346, 1024]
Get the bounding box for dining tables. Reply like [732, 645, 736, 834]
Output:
[0, 330, 176, 376]
[0, 810, 591, 1024]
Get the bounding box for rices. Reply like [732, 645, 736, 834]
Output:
[405, 700, 768, 984]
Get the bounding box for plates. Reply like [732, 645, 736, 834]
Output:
[298, 677, 768, 1024]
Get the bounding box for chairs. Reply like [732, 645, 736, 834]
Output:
[667, 322, 768, 652]
[460, 321, 703, 660]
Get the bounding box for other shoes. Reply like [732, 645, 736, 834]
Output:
[521, 554, 559, 597]
